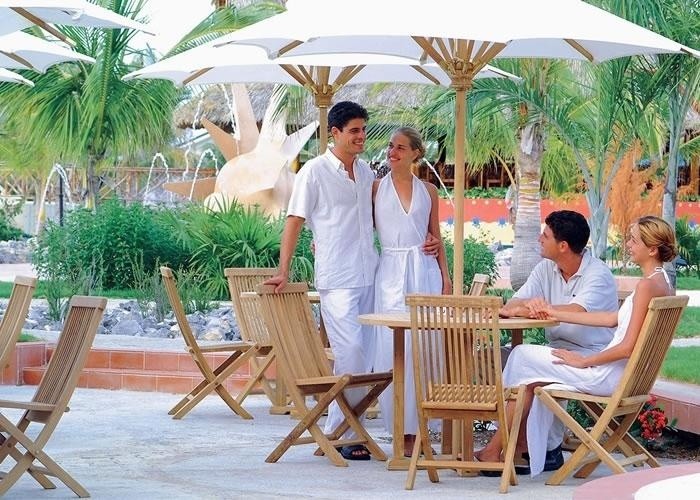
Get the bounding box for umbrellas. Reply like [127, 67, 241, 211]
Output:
[120, 23, 521, 159]
[0, 29, 96, 75]
[0, 66, 34, 86]
[214, 0, 699, 313]
[0, 4, 156, 48]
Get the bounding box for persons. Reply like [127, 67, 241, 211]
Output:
[452, 214, 678, 478]
[308, 127, 455, 457]
[257, 100, 440, 461]
[474, 209, 620, 472]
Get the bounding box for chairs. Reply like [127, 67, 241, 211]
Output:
[0, 276, 106, 500]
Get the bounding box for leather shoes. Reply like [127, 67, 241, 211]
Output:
[529, 447, 565, 472]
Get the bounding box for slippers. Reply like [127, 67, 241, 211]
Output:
[458, 453, 502, 477]
[341, 445, 371, 460]
